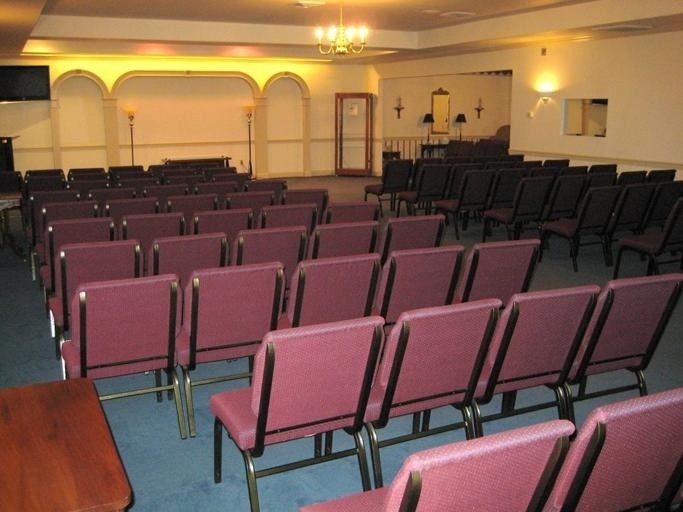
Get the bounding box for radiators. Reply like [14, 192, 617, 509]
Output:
[383, 136, 480, 165]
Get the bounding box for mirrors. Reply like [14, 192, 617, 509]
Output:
[431, 87, 450, 134]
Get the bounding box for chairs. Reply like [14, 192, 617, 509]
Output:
[550, 386, 682, 511]
[299, 420, 575, 510]
[508, 273, 682, 425]
[23, 155, 542, 438]
[362, 156, 682, 282]
[325, 299, 503, 489]
[209, 315, 384, 511]
[422, 286, 602, 442]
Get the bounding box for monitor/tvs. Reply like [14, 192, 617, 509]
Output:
[0, 66, 50, 100]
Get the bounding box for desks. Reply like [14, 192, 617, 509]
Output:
[421, 145, 448, 159]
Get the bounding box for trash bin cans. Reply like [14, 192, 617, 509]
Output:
[382, 151, 400, 173]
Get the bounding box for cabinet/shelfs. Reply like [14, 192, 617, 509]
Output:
[335, 92, 373, 177]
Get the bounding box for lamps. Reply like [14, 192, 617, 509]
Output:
[455, 114, 466, 140]
[313, 4, 369, 55]
[422, 114, 435, 144]
[122, 103, 140, 166]
[243, 105, 256, 180]
